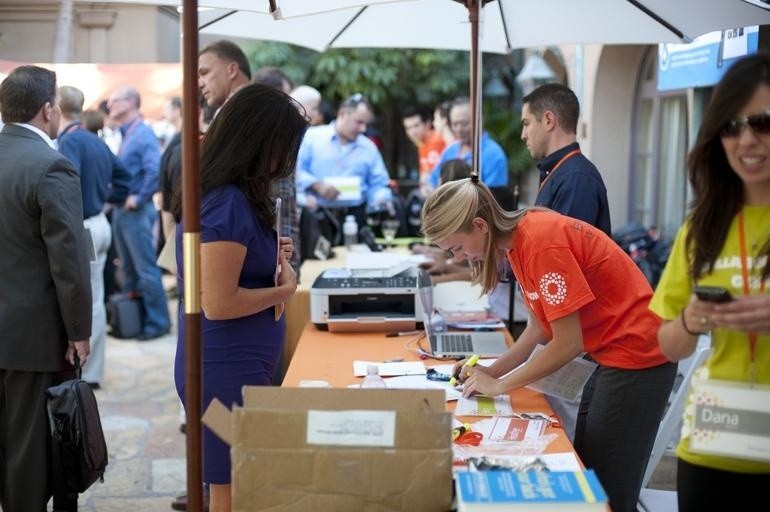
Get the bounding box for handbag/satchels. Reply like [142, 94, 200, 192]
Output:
[44, 349, 112, 497]
[106, 288, 148, 340]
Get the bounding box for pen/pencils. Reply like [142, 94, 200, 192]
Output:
[386, 330, 420, 337]
[384, 358, 404, 363]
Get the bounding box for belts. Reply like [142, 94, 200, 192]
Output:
[316, 201, 367, 216]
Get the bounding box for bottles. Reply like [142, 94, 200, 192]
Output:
[343, 215, 357, 246]
[359, 365, 387, 388]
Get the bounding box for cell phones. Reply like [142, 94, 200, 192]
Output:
[693, 287, 731, 302]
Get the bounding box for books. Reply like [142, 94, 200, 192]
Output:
[453, 464, 612, 512]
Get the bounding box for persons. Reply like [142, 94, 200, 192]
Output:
[172, 83, 313, 510]
[646, 52, 770, 510]
[0, 65, 94, 511]
[171, 38, 301, 510]
[519, 83, 612, 444]
[417, 172, 679, 511]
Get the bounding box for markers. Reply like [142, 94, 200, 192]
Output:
[447, 352, 481, 387]
[451, 422, 470, 441]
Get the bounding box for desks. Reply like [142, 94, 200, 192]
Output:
[283, 230, 613, 511]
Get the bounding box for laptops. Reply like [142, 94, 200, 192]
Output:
[416, 270, 510, 359]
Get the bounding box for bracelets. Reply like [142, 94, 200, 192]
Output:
[681, 308, 705, 336]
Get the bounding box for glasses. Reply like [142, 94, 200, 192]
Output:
[718, 111, 770, 138]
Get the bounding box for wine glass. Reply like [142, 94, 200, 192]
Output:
[381, 220, 398, 249]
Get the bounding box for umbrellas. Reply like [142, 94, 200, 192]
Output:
[179, 0, 769, 182]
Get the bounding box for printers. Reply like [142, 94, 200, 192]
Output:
[311, 268, 433, 333]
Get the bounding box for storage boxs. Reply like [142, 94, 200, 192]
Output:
[200, 381, 453, 511]
